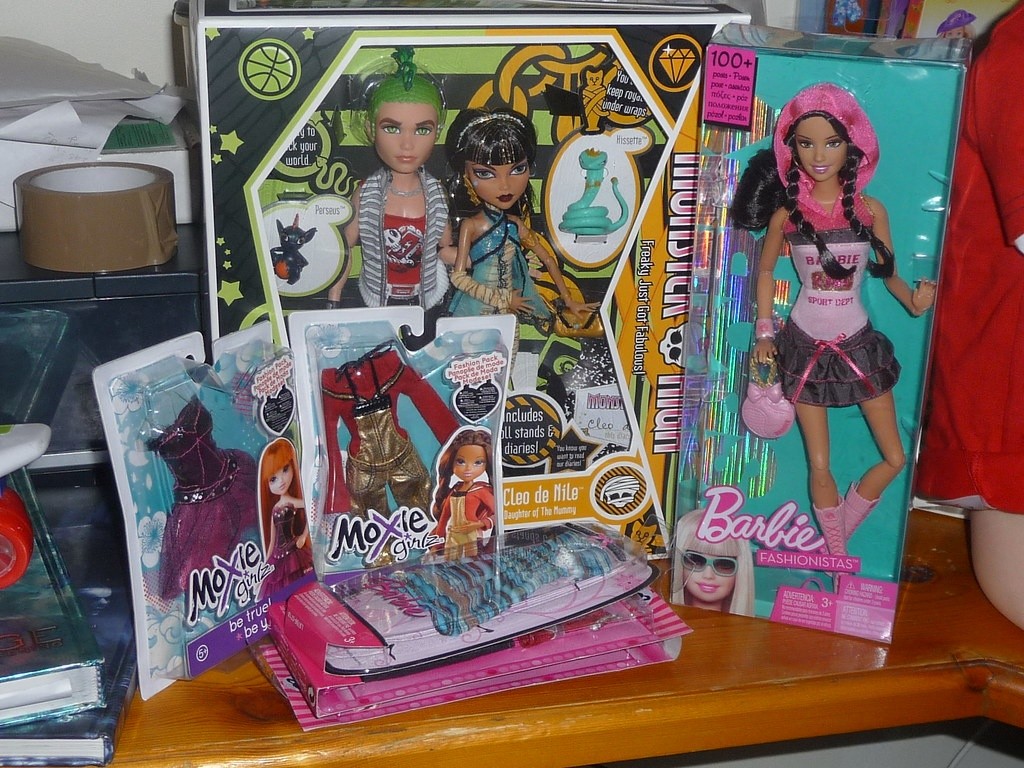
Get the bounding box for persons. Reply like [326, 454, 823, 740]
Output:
[730, 84, 938, 597]
[443, 105, 600, 317]
[328, 46, 474, 350]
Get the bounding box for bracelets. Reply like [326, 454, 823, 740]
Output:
[755, 318, 776, 341]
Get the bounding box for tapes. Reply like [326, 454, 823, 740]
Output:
[13, 161, 179, 272]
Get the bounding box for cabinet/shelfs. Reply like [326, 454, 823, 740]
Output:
[1, 221, 209, 643]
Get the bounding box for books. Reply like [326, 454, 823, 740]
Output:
[0, 464, 136, 765]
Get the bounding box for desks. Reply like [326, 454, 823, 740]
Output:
[81, 508, 1024, 768]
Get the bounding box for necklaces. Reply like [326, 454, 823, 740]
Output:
[390, 186, 422, 198]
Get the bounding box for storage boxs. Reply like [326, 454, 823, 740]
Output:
[172, 0, 752, 560]
